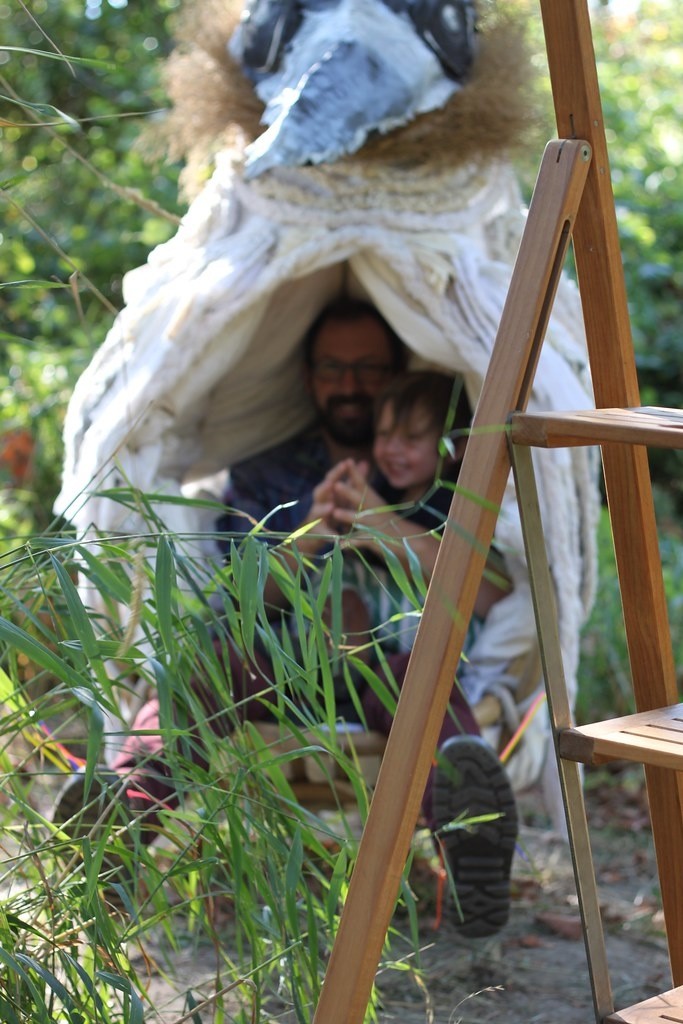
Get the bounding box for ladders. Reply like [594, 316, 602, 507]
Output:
[309, 0, 683, 1024]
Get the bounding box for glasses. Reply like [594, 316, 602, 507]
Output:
[312, 361, 395, 387]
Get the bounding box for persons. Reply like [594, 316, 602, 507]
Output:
[47, 295, 519, 940]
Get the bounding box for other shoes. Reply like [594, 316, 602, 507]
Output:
[319, 582, 370, 699]
[48, 764, 138, 923]
[425, 732, 521, 941]
